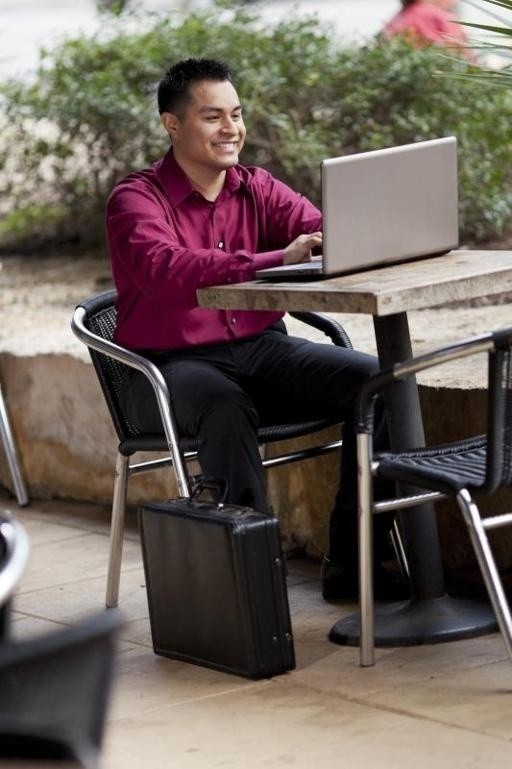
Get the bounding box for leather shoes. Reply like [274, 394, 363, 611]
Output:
[317, 554, 413, 605]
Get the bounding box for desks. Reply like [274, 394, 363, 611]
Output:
[197, 251, 512, 647]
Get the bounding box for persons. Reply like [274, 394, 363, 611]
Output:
[376, 0, 476, 65]
[104, 57, 413, 605]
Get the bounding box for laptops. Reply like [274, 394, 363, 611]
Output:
[255, 136, 460, 281]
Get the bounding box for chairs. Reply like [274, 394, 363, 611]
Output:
[0, 614, 129, 769]
[72, 289, 408, 610]
[0, 509, 29, 638]
[0, 391, 29, 506]
[358, 328, 512, 667]
